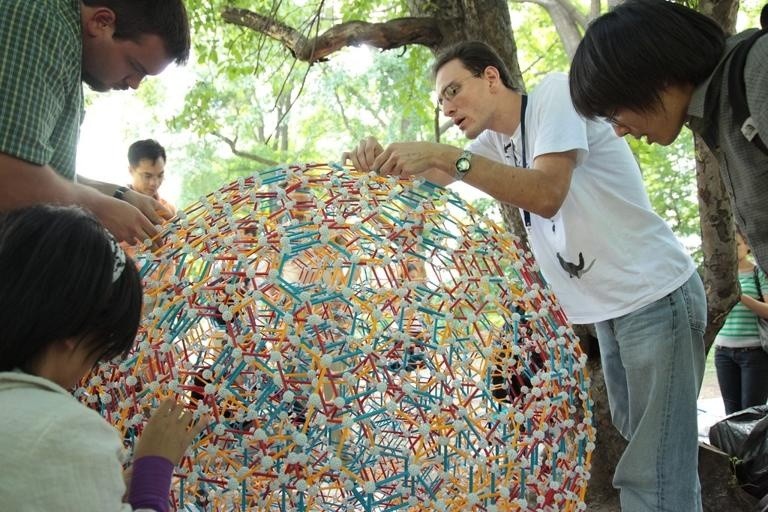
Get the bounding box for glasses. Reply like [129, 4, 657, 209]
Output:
[439, 73, 476, 107]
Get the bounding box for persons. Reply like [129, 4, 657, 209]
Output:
[713, 230, 768, 418]
[189, 225, 311, 436]
[339, 42, 707, 512]
[127, 140, 176, 222]
[569, 1, 767, 277]
[487, 303, 543, 436]
[0, 202, 210, 512]
[385, 264, 426, 372]
[0, 0, 191, 260]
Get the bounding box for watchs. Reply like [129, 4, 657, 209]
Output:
[453, 149, 473, 183]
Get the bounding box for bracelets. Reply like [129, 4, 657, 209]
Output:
[127, 455, 174, 512]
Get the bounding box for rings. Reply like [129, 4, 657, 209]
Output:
[113, 187, 129, 199]
